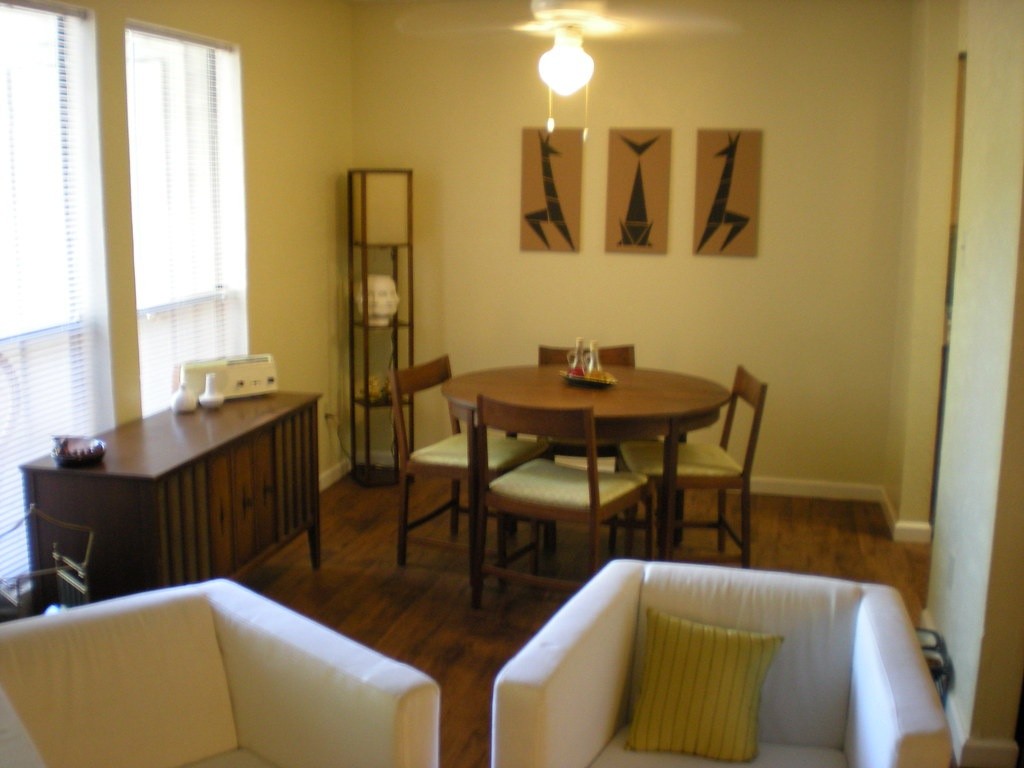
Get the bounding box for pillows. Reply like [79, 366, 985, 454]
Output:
[624, 609, 781, 762]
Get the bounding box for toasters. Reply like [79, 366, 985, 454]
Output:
[178, 352, 278, 402]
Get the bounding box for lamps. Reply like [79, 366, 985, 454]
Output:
[537, 23, 594, 142]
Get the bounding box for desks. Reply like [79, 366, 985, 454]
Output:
[441, 366, 734, 599]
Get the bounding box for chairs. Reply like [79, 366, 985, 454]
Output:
[613, 366, 767, 561]
[537, 345, 656, 552]
[386, 352, 545, 583]
[473, 391, 650, 602]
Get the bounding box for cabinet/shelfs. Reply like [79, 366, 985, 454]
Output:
[347, 168, 416, 487]
[19, 390, 324, 618]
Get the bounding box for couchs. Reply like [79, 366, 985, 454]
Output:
[490, 559, 953, 768]
[0, 579, 440, 768]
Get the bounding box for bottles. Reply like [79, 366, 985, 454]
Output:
[171, 371, 225, 414]
[566, 337, 605, 380]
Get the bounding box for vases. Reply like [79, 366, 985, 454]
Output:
[198, 373, 224, 411]
[171, 383, 197, 412]
